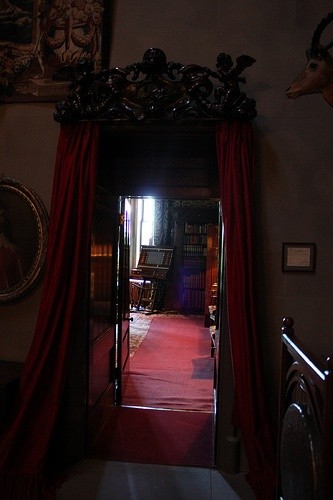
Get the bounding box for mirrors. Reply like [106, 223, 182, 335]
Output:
[52, 48, 265, 467]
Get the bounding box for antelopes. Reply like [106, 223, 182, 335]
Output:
[286, 13, 333, 107]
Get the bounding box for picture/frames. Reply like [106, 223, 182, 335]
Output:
[281, 241, 316, 272]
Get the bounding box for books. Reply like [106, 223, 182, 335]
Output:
[182, 215, 206, 256]
[183, 271, 206, 310]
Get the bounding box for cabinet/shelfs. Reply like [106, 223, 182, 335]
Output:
[176, 219, 208, 314]
[127, 246, 175, 313]
